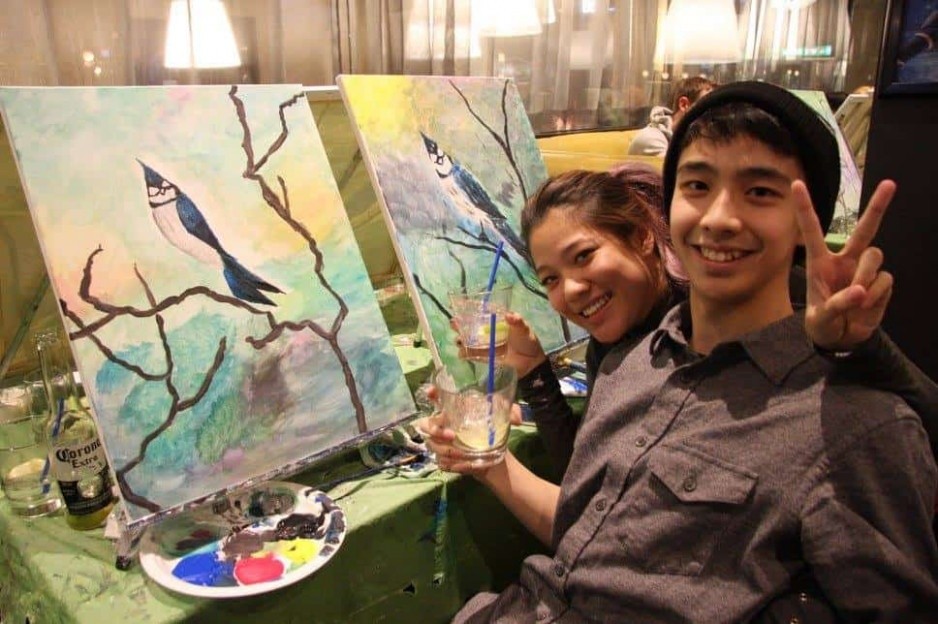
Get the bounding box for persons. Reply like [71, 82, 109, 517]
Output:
[422, 81, 938, 624]
[825, 85, 874, 114]
[449, 163, 938, 474]
[627, 76, 720, 158]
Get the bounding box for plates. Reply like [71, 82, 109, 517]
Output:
[137, 480, 347, 598]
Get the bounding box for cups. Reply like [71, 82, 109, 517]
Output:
[447, 283, 514, 349]
[24, 366, 77, 446]
[432, 356, 519, 467]
[0, 380, 62, 520]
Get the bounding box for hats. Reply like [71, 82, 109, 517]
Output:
[662, 81, 841, 264]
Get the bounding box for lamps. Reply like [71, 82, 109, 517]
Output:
[653, 0, 742, 63]
[162, 0, 243, 70]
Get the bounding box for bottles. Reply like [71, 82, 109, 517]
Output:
[35, 327, 116, 530]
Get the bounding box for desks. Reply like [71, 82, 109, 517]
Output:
[1, 293, 591, 624]
